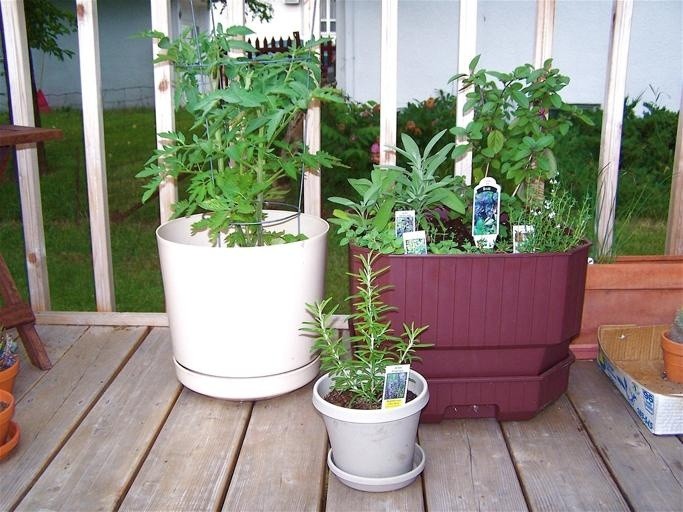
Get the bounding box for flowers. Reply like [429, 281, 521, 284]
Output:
[0, 321, 18, 367]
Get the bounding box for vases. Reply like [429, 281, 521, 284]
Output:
[0, 352, 20, 461]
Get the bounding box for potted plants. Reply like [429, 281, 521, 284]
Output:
[658, 305, 683, 385]
